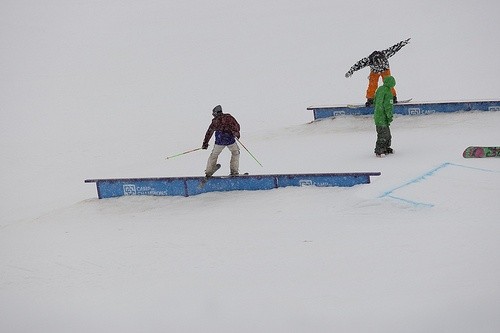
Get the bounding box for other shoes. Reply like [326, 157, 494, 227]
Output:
[366, 100, 373, 106]
[393, 97, 396, 103]
[386, 148, 393, 154]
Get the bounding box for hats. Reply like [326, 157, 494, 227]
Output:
[213, 105, 222, 115]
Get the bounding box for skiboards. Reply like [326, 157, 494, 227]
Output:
[197, 163, 248, 190]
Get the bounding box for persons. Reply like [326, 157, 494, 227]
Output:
[202, 105, 241, 177]
[345, 38, 410, 106]
[373, 75, 396, 156]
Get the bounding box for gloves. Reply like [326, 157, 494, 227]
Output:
[202, 142, 209, 149]
[405, 37, 411, 44]
[345, 72, 352, 78]
[234, 133, 241, 139]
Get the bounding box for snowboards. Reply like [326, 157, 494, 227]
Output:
[463, 146, 500, 158]
[347, 97, 413, 109]
